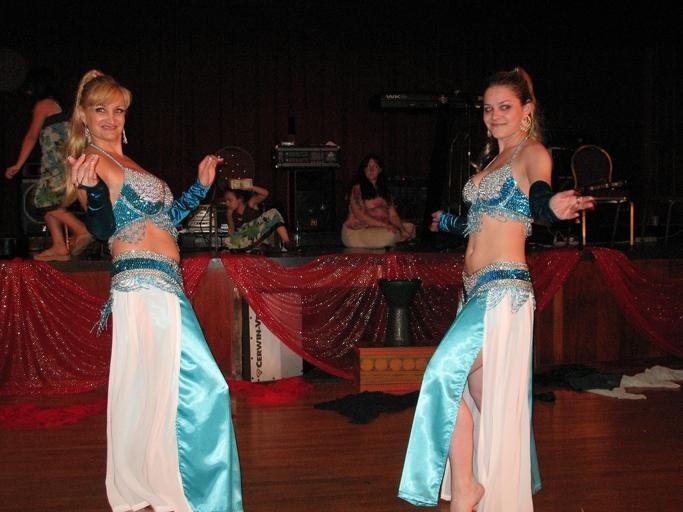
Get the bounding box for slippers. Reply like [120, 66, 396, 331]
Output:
[35, 251, 69, 262]
[70, 233, 94, 256]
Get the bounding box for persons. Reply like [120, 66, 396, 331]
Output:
[341, 155, 417, 249]
[66, 69, 244, 512]
[5, 94, 93, 263]
[397, 66, 595, 512]
[223, 184, 290, 253]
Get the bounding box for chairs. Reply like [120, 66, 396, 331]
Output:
[209, 145, 256, 250]
[570, 145, 635, 247]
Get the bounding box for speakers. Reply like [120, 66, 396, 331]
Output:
[274, 166, 337, 232]
[18, 162, 53, 238]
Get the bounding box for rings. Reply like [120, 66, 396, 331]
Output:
[576, 196, 581, 205]
